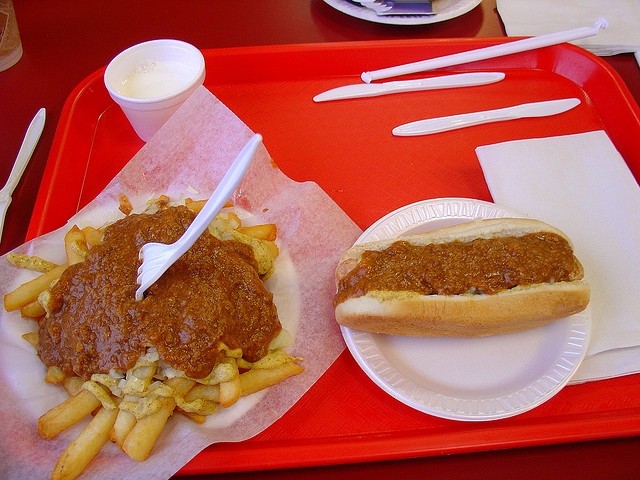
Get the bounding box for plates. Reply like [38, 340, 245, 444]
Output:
[324, 0, 485, 25]
[334, 198, 591, 424]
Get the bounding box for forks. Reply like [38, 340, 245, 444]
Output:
[0, 106, 47, 243]
[134, 131, 263, 301]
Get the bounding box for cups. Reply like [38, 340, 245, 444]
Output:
[0, 34, 640, 480]
[0, 0, 23, 74]
[103, 37, 206, 146]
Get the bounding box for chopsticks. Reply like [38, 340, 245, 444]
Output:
[366, 24, 597, 80]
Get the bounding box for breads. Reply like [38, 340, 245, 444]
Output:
[330, 216, 591, 337]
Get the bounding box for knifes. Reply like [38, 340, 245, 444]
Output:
[314, 70, 503, 104]
[392, 95, 580, 138]
